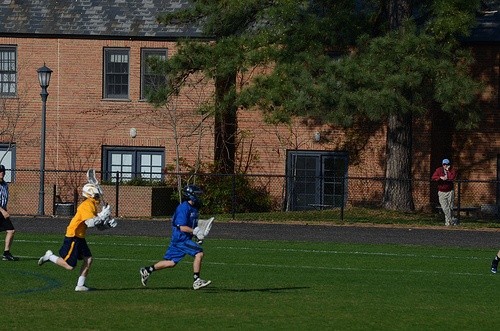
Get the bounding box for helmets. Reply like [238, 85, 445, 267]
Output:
[81, 183, 100, 204]
[181, 185, 204, 203]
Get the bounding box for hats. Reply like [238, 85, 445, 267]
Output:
[442, 159, 450, 165]
[0, 165, 5, 172]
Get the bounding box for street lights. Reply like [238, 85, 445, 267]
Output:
[35, 62, 54, 217]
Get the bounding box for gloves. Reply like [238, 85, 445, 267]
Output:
[102, 217, 118, 229]
[193, 227, 204, 244]
[97, 206, 111, 221]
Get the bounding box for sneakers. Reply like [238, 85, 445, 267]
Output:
[38, 250, 53, 265]
[74, 286, 89, 292]
[2, 255, 17, 261]
[140, 267, 150, 286]
[491, 261, 498, 274]
[193, 278, 212, 289]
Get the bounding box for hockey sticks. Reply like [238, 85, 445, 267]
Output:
[197, 217, 215, 237]
[87, 170, 112, 220]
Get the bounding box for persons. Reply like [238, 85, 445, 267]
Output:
[140, 184, 212, 290]
[491, 250, 500, 274]
[0, 164, 20, 261]
[432, 159, 457, 226]
[39, 184, 116, 291]
